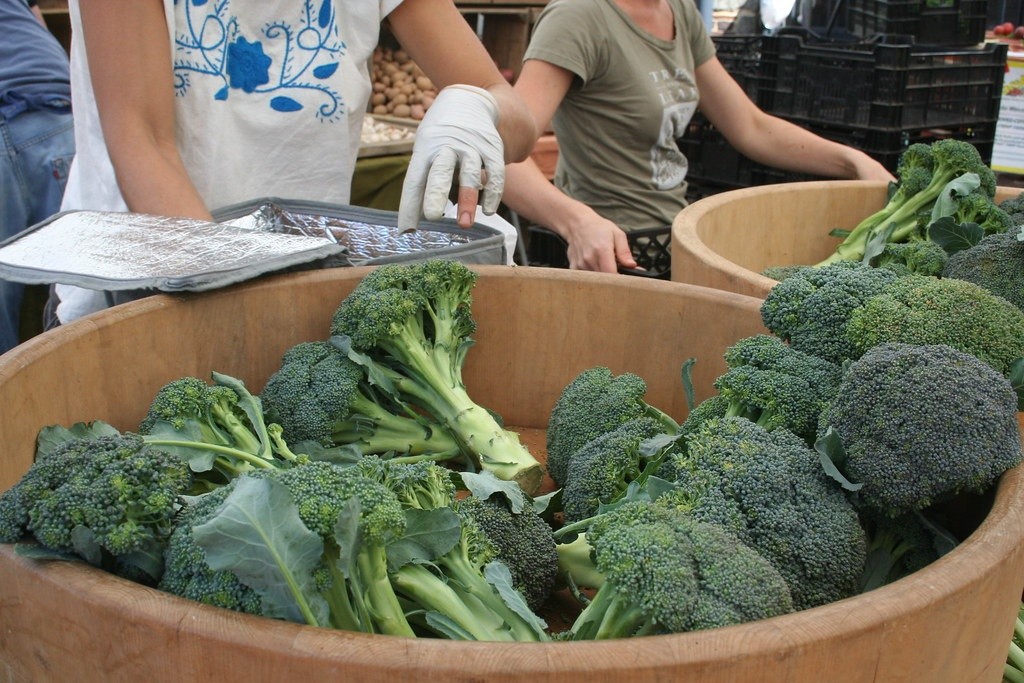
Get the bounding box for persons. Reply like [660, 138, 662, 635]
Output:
[486, 1, 902, 274]
[0, 0, 536, 369]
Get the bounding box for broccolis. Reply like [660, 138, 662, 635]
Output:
[0, 135, 1024, 643]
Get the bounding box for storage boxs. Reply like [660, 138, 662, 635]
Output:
[674, 0, 1010, 206]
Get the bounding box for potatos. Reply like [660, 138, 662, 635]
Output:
[363, 46, 439, 120]
